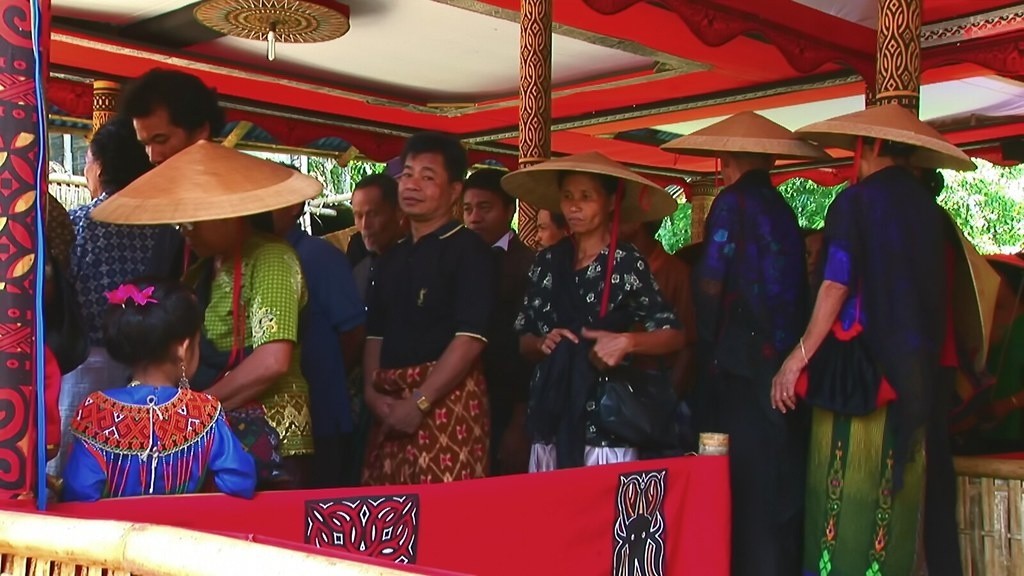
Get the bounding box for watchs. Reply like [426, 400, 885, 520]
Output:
[414, 389, 430, 413]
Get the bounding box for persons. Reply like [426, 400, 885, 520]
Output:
[87, 137, 323, 491]
[68, 116, 197, 362]
[793, 103, 1018, 575]
[499, 151, 678, 473]
[361, 129, 501, 487]
[660, 108, 834, 576]
[270, 155, 826, 491]
[59, 260, 259, 501]
[128, 65, 228, 299]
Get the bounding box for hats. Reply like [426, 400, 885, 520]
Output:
[793, 104, 977, 172]
[501, 152, 677, 222]
[661, 111, 834, 160]
[91, 140, 324, 224]
